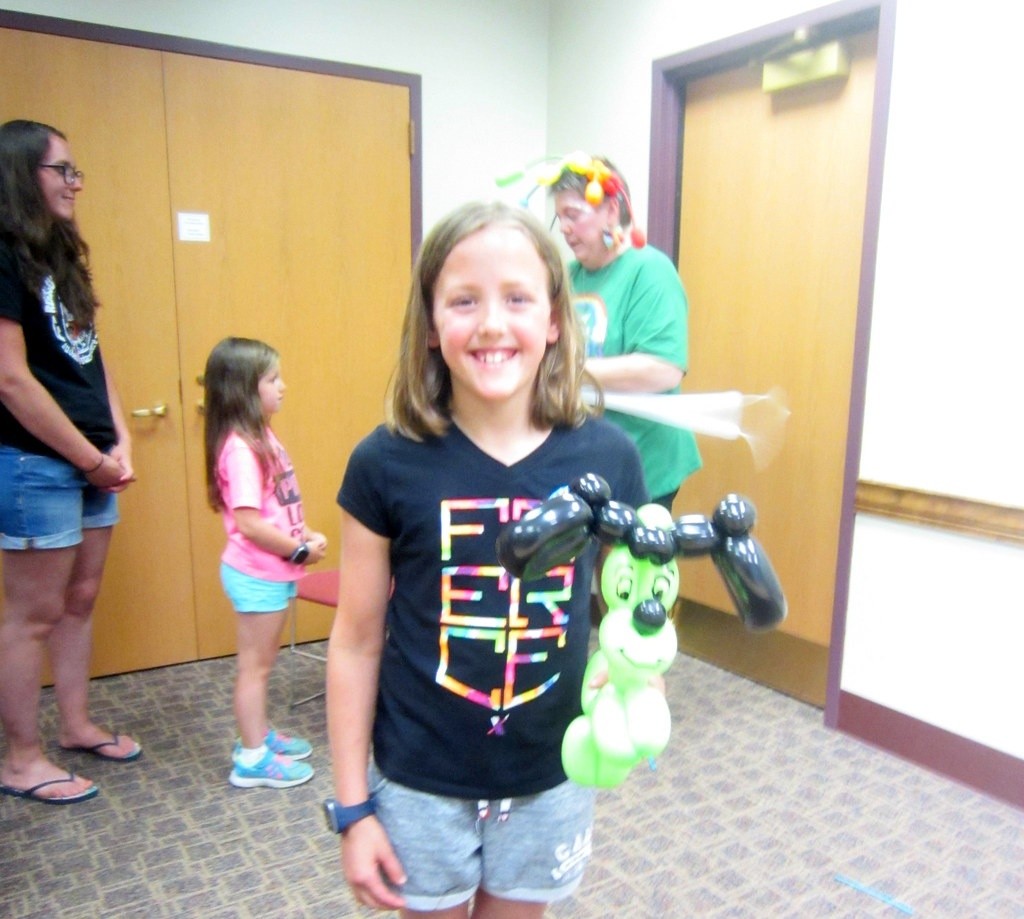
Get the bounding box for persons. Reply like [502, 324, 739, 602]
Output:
[322, 198, 666, 919]
[548, 161, 704, 619]
[0, 119, 140, 804]
[204, 336, 330, 789]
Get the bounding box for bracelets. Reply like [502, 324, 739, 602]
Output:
[85, 454, 103, 474]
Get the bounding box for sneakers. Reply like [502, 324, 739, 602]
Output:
[231, 728, 314, 760]
[228, 748, 315, 789]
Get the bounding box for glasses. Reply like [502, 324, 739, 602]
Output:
[40, 164, 85, 184]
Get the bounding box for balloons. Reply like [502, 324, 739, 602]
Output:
[497, 156, 645, 250]
[495, 474, 787, 789]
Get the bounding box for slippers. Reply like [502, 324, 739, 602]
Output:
[58, 729, 144, 762]
[0, 768, 100, 805]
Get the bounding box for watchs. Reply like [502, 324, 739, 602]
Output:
[282, 542, 309, 564]
[323, 799, 376, 835]
